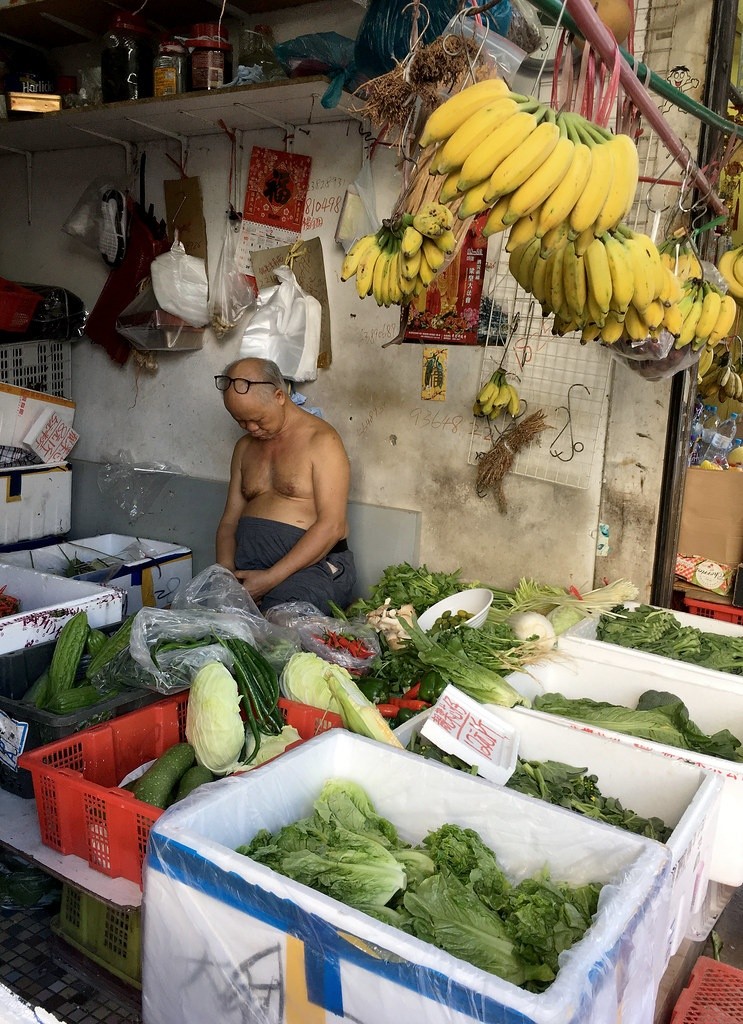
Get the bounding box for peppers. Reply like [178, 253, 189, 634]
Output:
[311, 627, 447, 729]
[103, 625, 288, 767]
[0, 584, 21, 618]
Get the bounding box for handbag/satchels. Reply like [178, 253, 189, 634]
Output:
[275, 32, 359, 108]
[206, 214, 256, 341]
[606, 327, 674, 361]
[611, 342, 707, 383]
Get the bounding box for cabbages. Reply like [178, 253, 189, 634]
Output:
[547, 606, 586, 633]
[183, 662, 303, 777]
[508, 610, 558, 650]
[278, 651, 352, 714]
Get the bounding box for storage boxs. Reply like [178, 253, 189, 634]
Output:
[392, 704, 724, 958]
[480, 649, 743, 889]
[48, 880, 141, 989]
[16, 692, 345, 885]
[0, 460, 72, 544]
[140, 728, 673, 1024]
[0, 382, 76, 451]
[667, 955, 743, 1024]
[0, 616, 190, 800]
[557, 601, 743, 694]
[0, 564, 123, 657]
[0, 533, 192, 618]
[6, 91, 61, 118]
[674, 463, 743, 596]
[0, 338, 71, 400]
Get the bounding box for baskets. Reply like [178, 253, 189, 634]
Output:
[49, 883, 143, 989]
[683, 597, 743, 626]
[670, 955, 743, 1024]
[0, 276, 44, 332]
[16, 688, 345, 894]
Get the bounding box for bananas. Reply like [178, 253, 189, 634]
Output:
[340, 202, 454, 308]
[420, 81, 743, 420]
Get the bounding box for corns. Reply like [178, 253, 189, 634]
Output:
[324, 670, 405, 751]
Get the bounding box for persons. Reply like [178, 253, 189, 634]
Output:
[198, 357, 358, 616]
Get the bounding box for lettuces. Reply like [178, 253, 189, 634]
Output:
[233, 779, 608, 993]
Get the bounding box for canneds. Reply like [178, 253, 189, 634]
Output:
[150, 39, 232, 96]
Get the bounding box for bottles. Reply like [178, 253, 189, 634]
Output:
[688, 405, 742, 470]
[99, 13, 234, 103]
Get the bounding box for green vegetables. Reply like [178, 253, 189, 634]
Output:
[596, 604, 743, 676]
[531, 690, 743, 765]
[396, 576, 637, 706]
[403, 730, 673, 844]
[328, 561, 479, 620]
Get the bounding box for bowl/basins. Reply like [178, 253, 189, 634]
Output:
[416, 588, 493, 635]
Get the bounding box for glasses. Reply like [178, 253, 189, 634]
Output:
[214, 375, 278, 394]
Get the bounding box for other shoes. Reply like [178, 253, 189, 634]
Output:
[98, 189, 131, 267]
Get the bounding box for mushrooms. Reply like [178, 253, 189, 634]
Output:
[366, 597, 418, 650]
